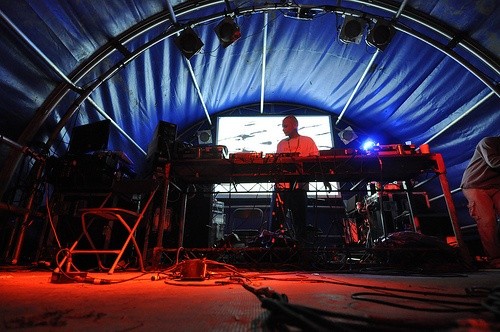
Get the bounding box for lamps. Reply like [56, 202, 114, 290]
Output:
[194, 129, 214, 144]
[363, 22, 399, 53]
[215, 13, 244, 46]
[338, 15, 365, 45]
[337, 127, 360, 145]
[175, 27, 206, 62]
[358, 136, 379, 151]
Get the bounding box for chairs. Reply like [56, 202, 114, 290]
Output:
[51, 175, 161, 275]
[228, 207, 265, 242]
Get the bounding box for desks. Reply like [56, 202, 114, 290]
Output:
[160, 152, 475, 273]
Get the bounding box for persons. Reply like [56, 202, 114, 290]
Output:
[271, 115, 320, 241]
[339, 179, 367, 223]
[460, 136, 500, 255]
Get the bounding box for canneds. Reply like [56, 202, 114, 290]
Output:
[398, 144, 402, 154]
[196, 147, 201, 159]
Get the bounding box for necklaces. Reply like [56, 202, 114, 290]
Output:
[288, 136, 299, 154]
